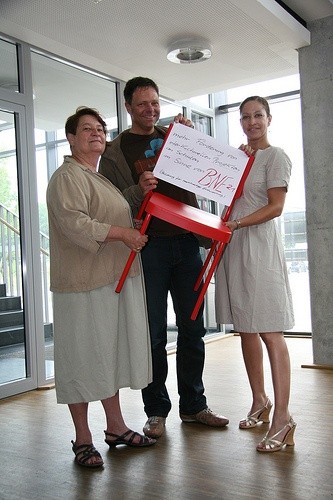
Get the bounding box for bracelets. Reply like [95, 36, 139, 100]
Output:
[234, 219, 242, 230]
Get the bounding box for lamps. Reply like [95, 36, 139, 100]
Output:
[165, 39, 212, 66]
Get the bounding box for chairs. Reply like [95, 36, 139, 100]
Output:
[115, 122, 255, 320]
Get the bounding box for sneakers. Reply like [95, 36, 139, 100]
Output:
[180, 408, 229, 426]
[143, 416, 166, 438]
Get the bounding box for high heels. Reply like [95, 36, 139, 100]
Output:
[256, 416, 297, 452]
[71, 440, 103, 468]
[239, 398, 273, 428]
[104, 429, 157, 450]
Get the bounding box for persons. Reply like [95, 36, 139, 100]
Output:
[46, 105, 157, 468]
[212, 96, 296, 453]
[98, 77, 229, 440]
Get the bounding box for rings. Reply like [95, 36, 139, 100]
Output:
[136, 247, 140, 250]
